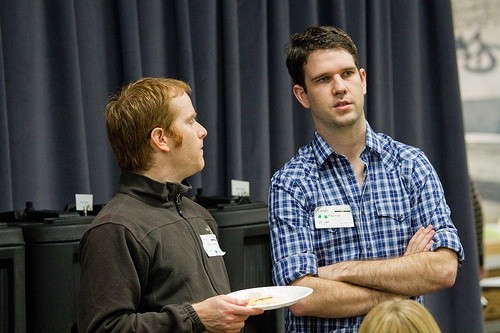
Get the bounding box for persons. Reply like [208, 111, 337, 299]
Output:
[78, 77, 264, 333]
[268, 25, 467, 333]
[356, 296, 443, 332]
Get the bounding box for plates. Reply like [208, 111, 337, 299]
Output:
[225, 285, 315, 311]
